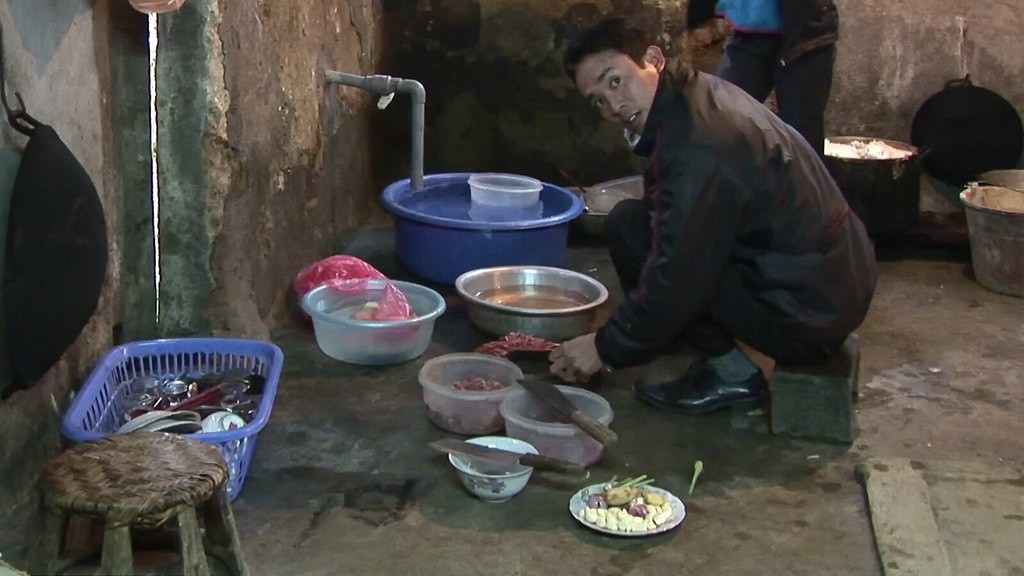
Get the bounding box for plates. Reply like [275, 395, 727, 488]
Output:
[112, 409, 203, 435]
[568, 481, 686, 536]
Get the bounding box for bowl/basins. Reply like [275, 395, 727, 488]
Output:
[417, 352, 525, 434]
[467, 171, 544, 207]
[377, 171, 584, 284]
[576, 175, 645, 233]
[448, 436, 540, 503]
[201, 411, 248, 433]
[455, 265, 609, 343]
[498, 384, 617, 466]
[300, 279, 447, 365]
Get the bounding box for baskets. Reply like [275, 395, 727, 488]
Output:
[59, 338, 284, 500]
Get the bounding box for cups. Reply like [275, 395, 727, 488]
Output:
[110, 368, 266, 422]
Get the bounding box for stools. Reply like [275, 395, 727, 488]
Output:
[771, 332, 864, 442]
[30, 432, 251, 575]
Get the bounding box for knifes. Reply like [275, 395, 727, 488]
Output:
[427, 436, 586, 477]
[516, 378, 618, 452]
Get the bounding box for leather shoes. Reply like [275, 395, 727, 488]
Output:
[641, 359, 770, 414]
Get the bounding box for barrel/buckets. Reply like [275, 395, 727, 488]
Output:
[823, 135, 921, 256]
[959, 179, 1024, 298]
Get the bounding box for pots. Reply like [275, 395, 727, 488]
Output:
[911, 78, 1024, 189]
[0, 108, 109, 400]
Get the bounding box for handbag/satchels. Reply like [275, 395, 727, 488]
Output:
[294, 254, 390, 322]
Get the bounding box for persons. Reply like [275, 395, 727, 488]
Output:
[713, 0, 839, 168]
[548, 18, 880, 414]
